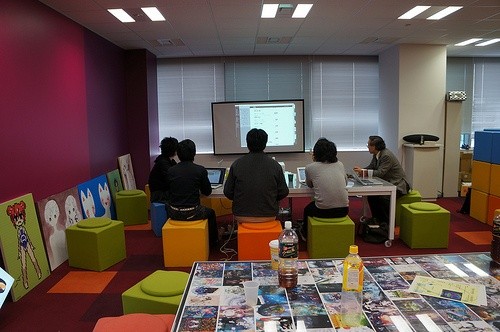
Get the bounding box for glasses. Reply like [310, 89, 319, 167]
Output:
[367, 144, 375, 147]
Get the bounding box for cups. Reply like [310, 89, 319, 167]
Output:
[361, 169, 367, 179]
[243, 281, 259, 305]
[269, 239, 279, 270]
[368, 170, 373, 179]
[288, 174, 293, 182]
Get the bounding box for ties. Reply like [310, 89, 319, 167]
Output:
[374, 156, 378, 168]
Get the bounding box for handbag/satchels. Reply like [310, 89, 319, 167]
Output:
[460, 187, 471, 215]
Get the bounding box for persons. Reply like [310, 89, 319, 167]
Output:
[223, 128, 289, 216]
[353, 136, 410, 231]
[148, 137, 178, 204]
[296, 138, 349, 243]
[164, 139, 223, 251]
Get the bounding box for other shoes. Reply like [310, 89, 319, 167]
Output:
[372, 228, 387, 235]
[299, 227, 308, 242]
[221, 232, 236, 243]
[211, 239, 223, 249]
[366, 217, 377, 225]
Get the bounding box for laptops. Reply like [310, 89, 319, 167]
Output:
[297, 167, 307, 185]
[205, 168, 226, 189]
[349, 169, 384, 185]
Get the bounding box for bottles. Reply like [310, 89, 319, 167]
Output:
[491, 209, 500, 265]
[278, 221, 298, 288]
[340, 245, 363, 326]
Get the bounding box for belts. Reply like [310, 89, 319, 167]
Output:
[169, 204, 199, 211]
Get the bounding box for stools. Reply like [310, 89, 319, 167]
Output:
[65, 190, 450, 332]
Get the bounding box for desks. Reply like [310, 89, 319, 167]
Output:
[169, 252, 500, 332]
[200, 177, 396, 247]
